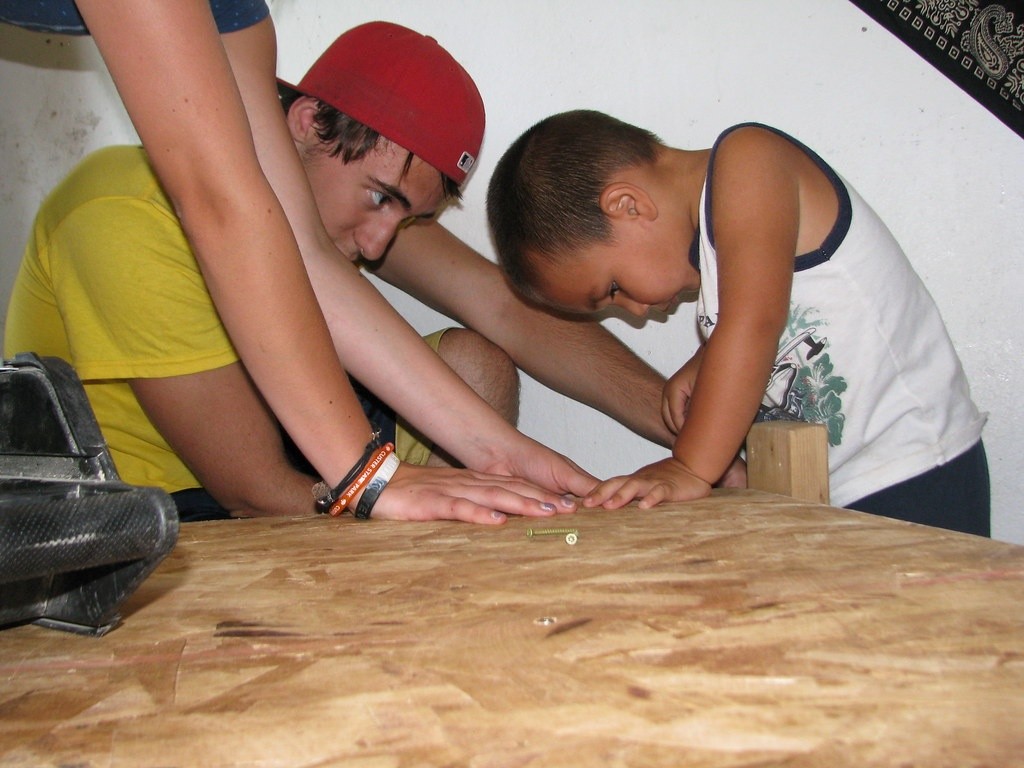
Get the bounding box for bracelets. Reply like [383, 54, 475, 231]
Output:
[310, 429, 398, 518]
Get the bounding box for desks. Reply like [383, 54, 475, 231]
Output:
[1, 434, 1024, 768]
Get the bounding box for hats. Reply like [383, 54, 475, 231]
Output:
[275, 21, 485, 187]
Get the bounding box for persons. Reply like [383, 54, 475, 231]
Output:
[2, 1, 604, 527]
[483, 109, 991, 540]
[0, 23, 754, 519]
